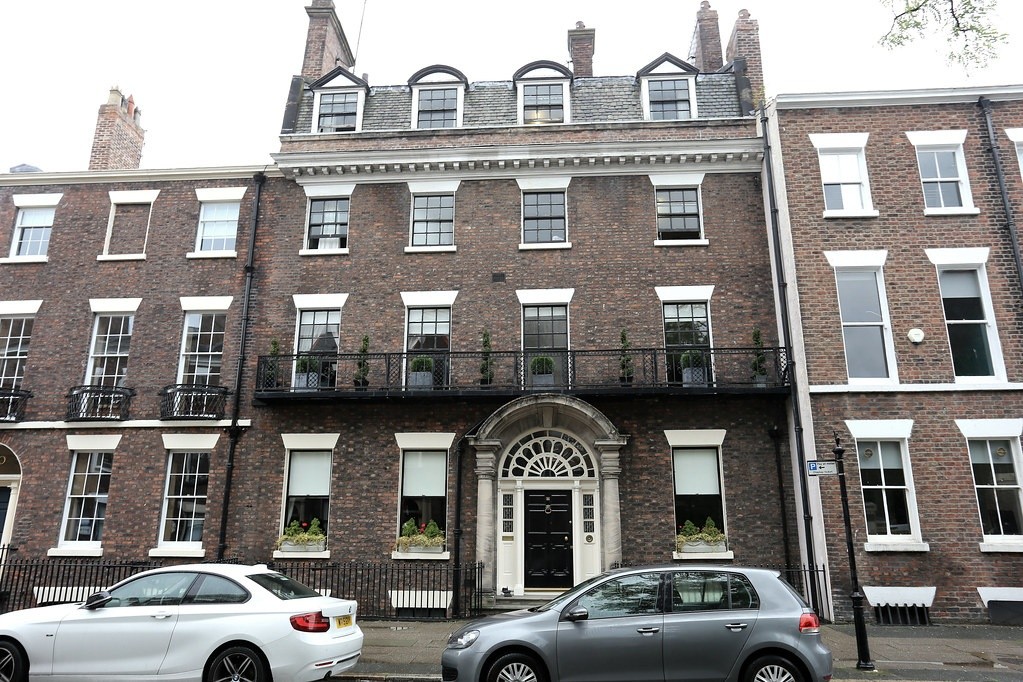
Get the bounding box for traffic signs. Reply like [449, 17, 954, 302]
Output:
[806, 461, 837, 476]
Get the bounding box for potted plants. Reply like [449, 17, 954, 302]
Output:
[264, 340, 281, 389]
[353, 335, 370, 393]
[407, 355, 433, 390]
[295, 357, 320, 393]
[675, 517, 726, 555]
[530, 357, 555, 386]
[750, 330, 767, 386]
[680, 350, 706, 389]
[480, 330, 496, 392]
[617, 327, 636, 387]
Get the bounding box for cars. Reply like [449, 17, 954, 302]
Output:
[0, 562, 366, 682]
[441, 559, 835, 682]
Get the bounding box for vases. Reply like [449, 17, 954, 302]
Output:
[399, 543, 443, 554]
[282, 542, 325, 551]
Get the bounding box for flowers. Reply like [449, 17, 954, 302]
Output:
[397, 517, 449, 552]
[272, 518, 325, 548]
